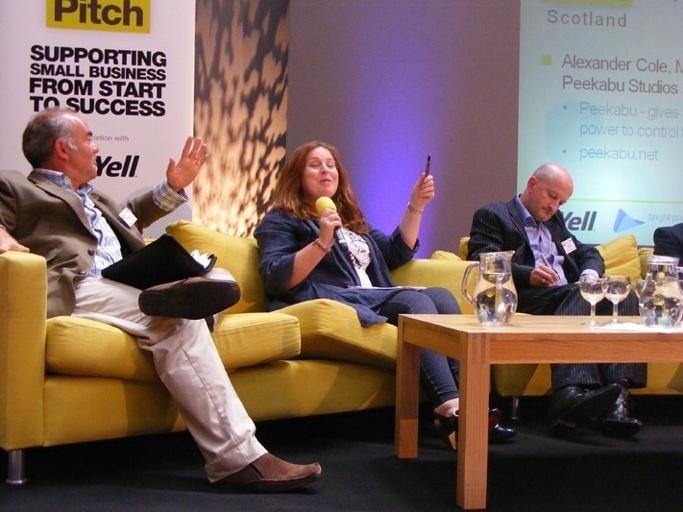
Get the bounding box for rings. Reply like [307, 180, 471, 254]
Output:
[542, 279, 545, 283]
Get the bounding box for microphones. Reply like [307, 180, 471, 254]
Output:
[315, 196, 348, 252]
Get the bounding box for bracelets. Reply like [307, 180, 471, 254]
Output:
[407, 200, 424, 215]
[313, 237, 330, 256]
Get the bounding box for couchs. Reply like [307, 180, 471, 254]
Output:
[0, 239, 487, 512]
[430, 250, 682, 421]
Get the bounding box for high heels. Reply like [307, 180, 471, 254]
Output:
[432, 406, 518, 451]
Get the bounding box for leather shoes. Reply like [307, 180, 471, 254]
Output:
[603, 389, 642, 437]
[545, 383, 622, 436]
[138, 277, 240, 321]
[212, 452, 322, 490]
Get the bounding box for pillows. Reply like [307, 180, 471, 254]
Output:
[165, 220, 271, 315]
[458, 236, 641, 289]
[638, 246, 655, 279]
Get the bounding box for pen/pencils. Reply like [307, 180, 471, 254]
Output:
[425, 155, 430, 176]
[541, 254, 552, 268]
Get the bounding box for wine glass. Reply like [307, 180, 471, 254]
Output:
[579, 273, 610, 328]
[599, 272, 632, 329]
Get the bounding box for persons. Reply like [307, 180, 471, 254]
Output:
[652, 222, 682, 285]
[0, 109, 322, 487]
[252, 139, 514, 449]
[470, 164, 647, 435]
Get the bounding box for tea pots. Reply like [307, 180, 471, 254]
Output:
[460, 249, 520, 329]
[632, 255, 683, 330]
